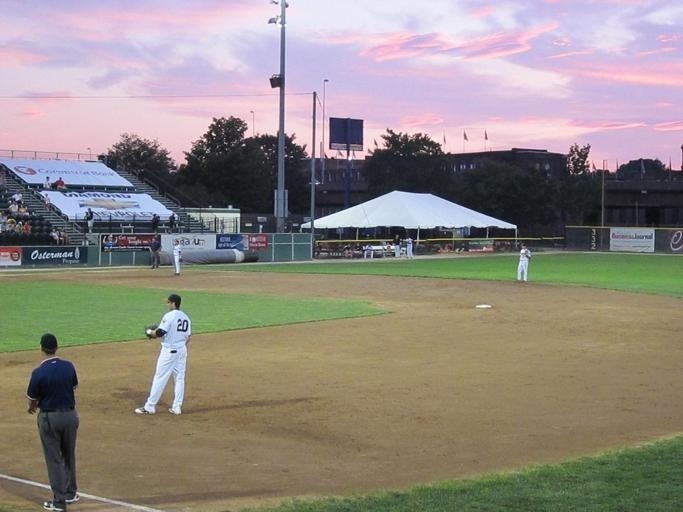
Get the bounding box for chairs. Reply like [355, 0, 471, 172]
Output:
[313, 243, 353, 257]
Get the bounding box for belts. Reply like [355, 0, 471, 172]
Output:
[41, 408, 73, 412]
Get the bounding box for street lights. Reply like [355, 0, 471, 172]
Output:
[86, 147, 91, 160]
[268, 0, 291, 232]
[320, 78, 329, 186]
[249, 110, 255, 137]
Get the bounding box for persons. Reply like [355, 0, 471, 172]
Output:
[0, 175, 67, 245]
[405, 235, 412, 258]
[342, 243, 353, 258]
[148, 236, 162, 268]
[173, 240, 183, 276]
[25, 333, 81, 511]
[393, 235, 401, 257]
[134, 294, 192, 416]
[518, 243, 531, 281]
[151, 214, 159, 233]
[169, 213, 176, 233]
[86, 207, 94, 233]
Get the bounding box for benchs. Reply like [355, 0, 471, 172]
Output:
[0, 169, 218, 245]
[363, 246, 406, 259]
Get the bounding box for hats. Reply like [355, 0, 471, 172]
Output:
[41, 334, 57, 350]
[163, 295, 181, 303]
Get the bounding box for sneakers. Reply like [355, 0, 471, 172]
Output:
[44, 501, 65, 512]
[135, 408, 155, 414]
[65, 494, 79, 503]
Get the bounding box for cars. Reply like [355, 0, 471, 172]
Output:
[366, 240, 385, 257]
